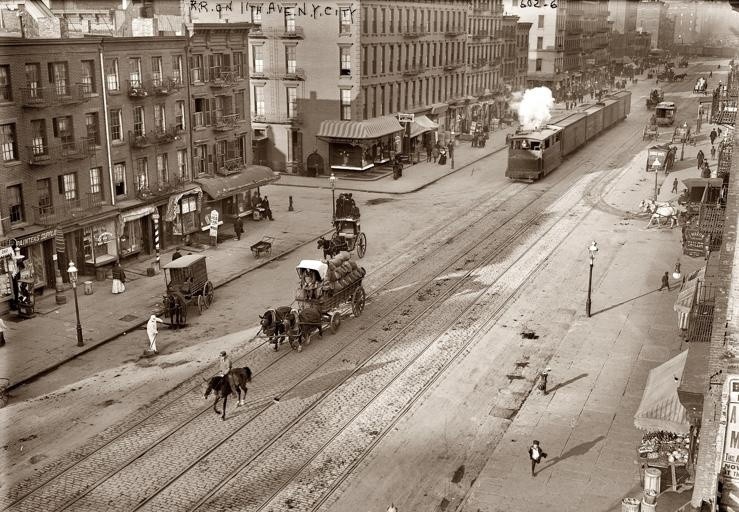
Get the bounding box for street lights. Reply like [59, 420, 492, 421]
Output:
[67, 259, 84, 347]
[651, 158, 662, 202]
[719, 79, 722, 102]
[594, 80, 597, 99]
[328, 173, 337, 227]
[678, 37, 684, 44]
[680, 122, 687, 161]
[450, 129, 455, 169]
[586, 240, 599, 317]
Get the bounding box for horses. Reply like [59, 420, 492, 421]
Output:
[202, 366, 252, 423]
[639, 198, 683, 230]
[161, 292, 184, 329]
[259, 306, 323, 353]
[316, 235, 347, 261]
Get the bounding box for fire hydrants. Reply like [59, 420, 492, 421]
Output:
[537, 371, 549, 397]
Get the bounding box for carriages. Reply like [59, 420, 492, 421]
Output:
[248, 251, 366, 352]
[642, 66, 707, 147]
[317, 193, 367, 260]
[163, 254, 214, 330]
[646, 141, 678, 174]
[638, 178, 723, 229]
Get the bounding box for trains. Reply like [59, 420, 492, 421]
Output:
[676, 44, 739, 58]
[505, 90, 632, 183]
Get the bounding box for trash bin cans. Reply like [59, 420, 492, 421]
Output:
[151, 262, 159, 274]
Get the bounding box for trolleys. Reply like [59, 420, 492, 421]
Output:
[0, 377, 10, 408]
[250, 236, 275, 259]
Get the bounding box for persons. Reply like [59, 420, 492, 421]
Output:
[251, 191, 258, 206]
[111, 262, 126, 295]
[423, 120, 488, 166]
[233, 217, 244, 240]
[528, 439, 547, 477]
[670, 178, 678, 193]
[172, 247, 182, 261]
[658, 271, 671, 291]
[217, 351, 239, 399]
[146, 314, 164, 352]
[301, 268, 317, 301]
[559, 53, 722, 179]
[262, 196, 274, 221]
[0, 318, 8, 346]
